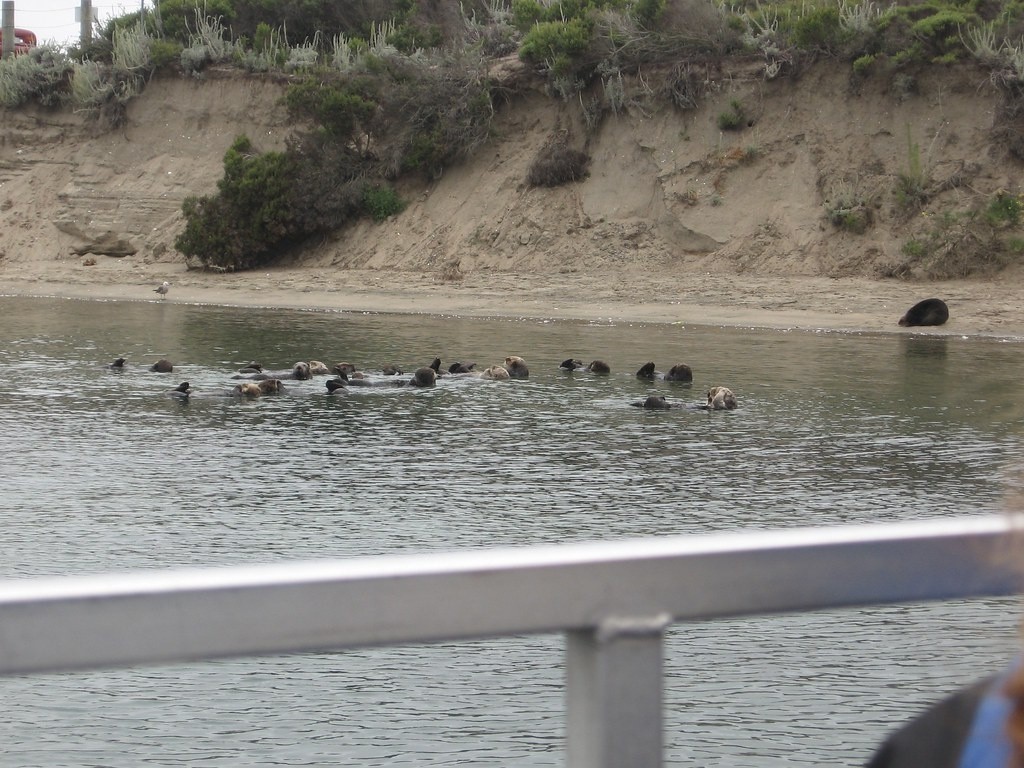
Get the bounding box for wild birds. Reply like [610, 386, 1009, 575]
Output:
[151, 281, 171, 301]
